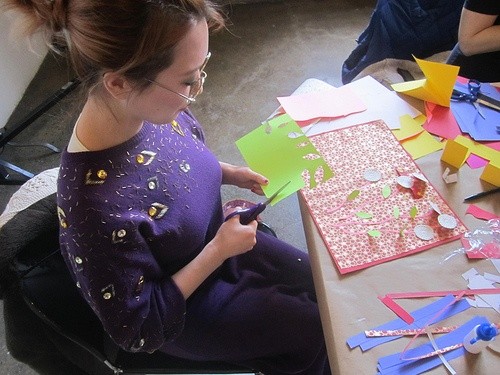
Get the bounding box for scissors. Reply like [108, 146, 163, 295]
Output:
[450, 79, 500, 120]
[224, 180, 292, 225]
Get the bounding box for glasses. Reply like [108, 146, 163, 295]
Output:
[100, 51, 211, 107]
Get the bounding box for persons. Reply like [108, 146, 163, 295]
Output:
[446, 0, 500, 83]
[12, 0, 331, 375]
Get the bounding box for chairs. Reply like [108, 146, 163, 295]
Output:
[0, 164, 263, 375]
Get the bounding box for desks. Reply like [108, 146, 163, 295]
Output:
[295, 81, 500, 375]
